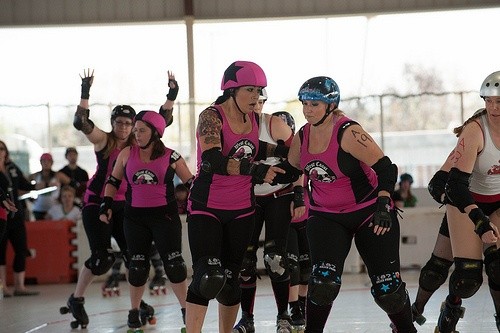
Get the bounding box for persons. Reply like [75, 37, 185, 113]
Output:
[392, 171, 418, 210]
[180, 62, 421, 333]
[432, 71, 500, 333]
[55, 147, 90, 197]
[42, 183, 84, 223]
[172, 182, 189, 216]
[389, 130, 484, 333]
[104, 231, 171, 297]
[99, 108, 201, 333]
[54, 69, 181, 329]
[0, 140, 42, 298]
[23, 152, 84, 221]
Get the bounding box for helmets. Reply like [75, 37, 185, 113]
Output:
[480, 71, 500, 96]
[298, 76, 340, 108]
[272, 111, 295, 133]
[221, 61, 267, 89]
[400, 173, 413, 183]
[133, 111, 167, 138]
[258, 88, 268, 101]
[112, 105, 136, 120]
[64, 148, 78, 157]
[41, 153, 52, 160]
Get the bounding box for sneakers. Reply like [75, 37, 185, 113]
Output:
[233, 312, 255, 333]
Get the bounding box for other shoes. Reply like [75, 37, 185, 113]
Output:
[277, 301, 306, 333]
[2, 287, 39, 297]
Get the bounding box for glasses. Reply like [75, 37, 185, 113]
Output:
[0, 148, 5, 150]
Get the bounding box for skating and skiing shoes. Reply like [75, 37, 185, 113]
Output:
[390, 303, 426, 333]
[435, 303, 465, 333]
[60, 292, 89, 329]
[102, 276, 120, 297]
[150, 276, 167, 296]
[126, 300, 155, 333]
[181, 307, 186, 333]
